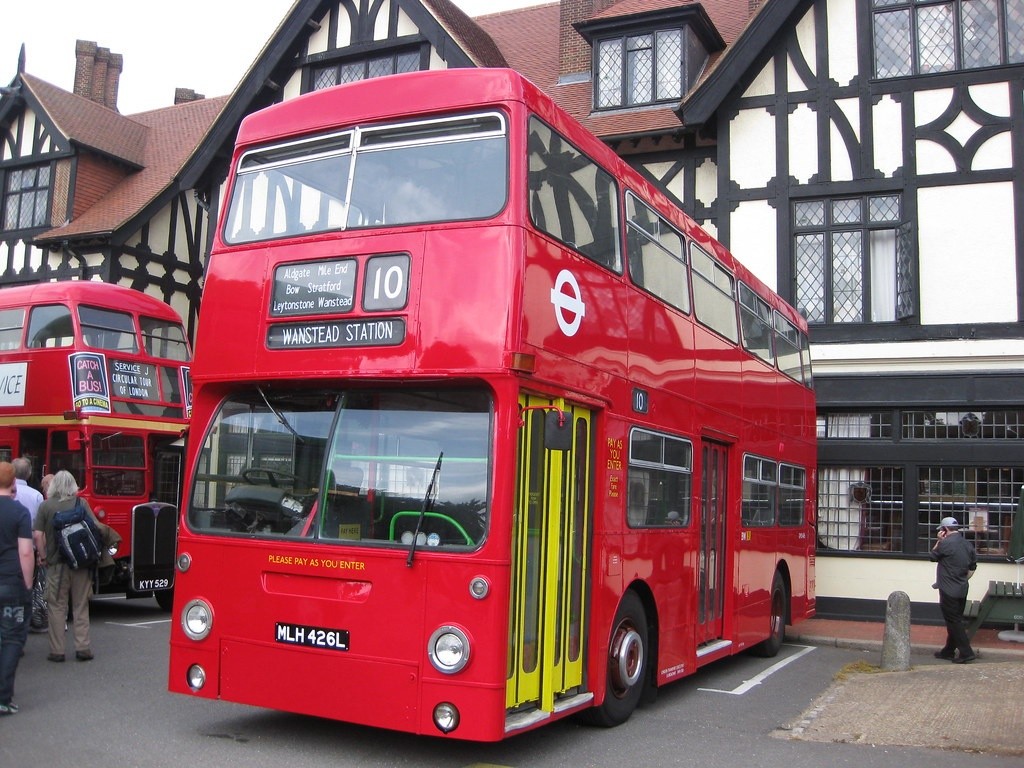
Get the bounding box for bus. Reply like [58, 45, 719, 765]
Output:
[1, 279, 195, 619]
[163, 63, 820, 749]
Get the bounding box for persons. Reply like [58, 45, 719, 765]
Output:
[33, 470, 100, 661]
[42, 473, 56, 499]
[11, 457, 45, 658]
[929, 515, 979, 662]
[0, 460, 36, 715]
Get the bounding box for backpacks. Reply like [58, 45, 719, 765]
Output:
[56, 495, 103, 599]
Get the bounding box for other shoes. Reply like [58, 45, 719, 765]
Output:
[934, 651, 952, 660]
[952, 655, 976, 664]
[76, 655, 96, 661]
[47, 653, 65, 664]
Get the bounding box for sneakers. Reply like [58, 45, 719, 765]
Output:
[27, 626, 49, 633]
[0, 702, 19, 715]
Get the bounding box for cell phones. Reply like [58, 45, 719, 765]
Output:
[942, 527, 947, 534]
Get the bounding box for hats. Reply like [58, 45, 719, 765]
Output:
[935, 516, 959, 530]
